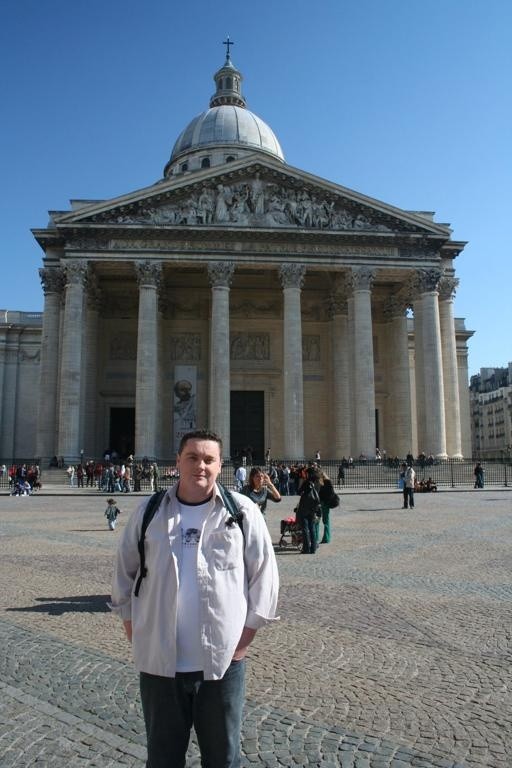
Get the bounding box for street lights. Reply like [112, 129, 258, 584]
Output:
[79, 448, 85, 465]
[381, 449, 387, 465]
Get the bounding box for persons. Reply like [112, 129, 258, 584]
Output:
[337, 449, 367, 485]
[104, 429, 279, 768]
[0, 464, 41, 497]
[474, 462, 484, 488]
[233, 444, 334, 554]
[373, 447, 438, 510]
[174, 380, 195, 420]
[104, 498, 122, 531]
[148, 172, 352, 229]
[66, 446, 161, 493]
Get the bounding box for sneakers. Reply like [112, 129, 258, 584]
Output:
[9, 493, 29, 497]
[67, 484, 161, 493]
[300, 541, 331, 554]
[402, 506, 415, 509]
[110, 528, 115, 531]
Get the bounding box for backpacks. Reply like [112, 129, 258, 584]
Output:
[301, 479, 320, 509]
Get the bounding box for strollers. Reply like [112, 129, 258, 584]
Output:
[277, 503, 307, 551]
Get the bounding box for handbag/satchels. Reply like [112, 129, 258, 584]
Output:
[321, 494, 340, 508]
[399, 479, 404, 488]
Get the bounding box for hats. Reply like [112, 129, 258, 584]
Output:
[106, 498, 117, 504]
